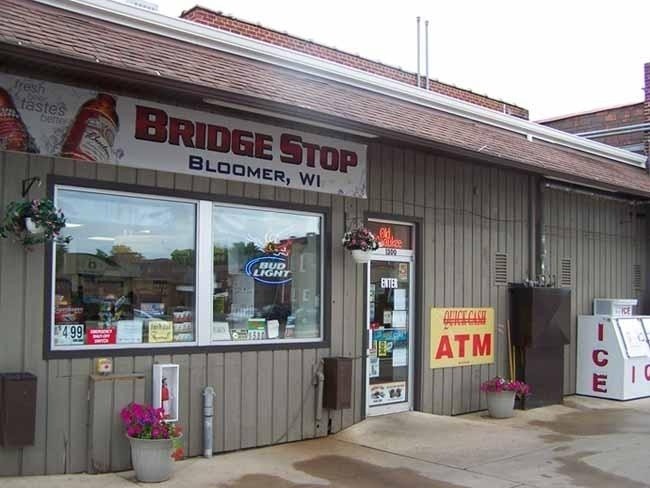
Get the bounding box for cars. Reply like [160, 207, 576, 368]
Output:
[251, 304, 291, 324]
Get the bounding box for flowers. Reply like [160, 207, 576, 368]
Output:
[340, 226, 379, 251]
[1, 193, 74, 246]
[120, 401, 187, 461]
[479, 374, 530, 397]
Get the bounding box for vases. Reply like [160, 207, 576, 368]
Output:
[124, 432, 183, 484]
[23, 217, 50, 236]
[351, 249, 372, 263]
[486, 391, 516, 419]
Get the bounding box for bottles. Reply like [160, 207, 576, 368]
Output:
[0, 87, 40, 153]
[61, 92, 121, 161]
